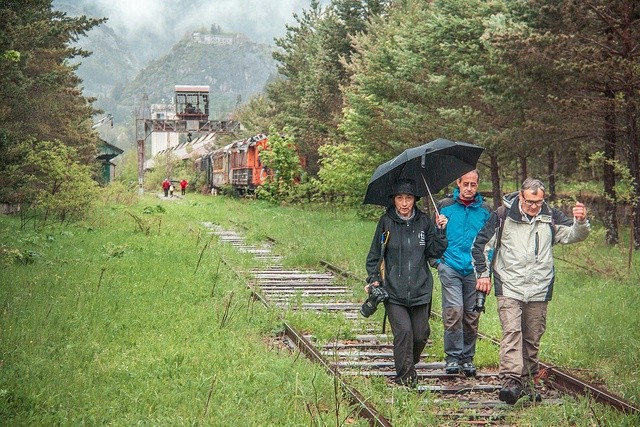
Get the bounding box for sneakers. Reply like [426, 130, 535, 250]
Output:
[500, 379, 521, 405]
[522, 387, 541, 402]
[460, 363, 476, 374]
[395, 369, 418, 386]
[446, 362, 462, 373]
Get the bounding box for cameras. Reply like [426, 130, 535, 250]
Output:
[360, 285, 390, 318]
[473, 290, 486, 314]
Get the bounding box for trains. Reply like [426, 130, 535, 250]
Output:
[194, 132, 306, 195]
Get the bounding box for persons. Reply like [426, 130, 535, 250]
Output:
[365, 177, 448, 388]
[430, 168, 497, 374]
[179, 179, 188, 195]
[169, 183, 175, 197]
[162, 178, 170, 197]
[471, 176, 591, 403]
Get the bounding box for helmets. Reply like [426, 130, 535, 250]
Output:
[387, 178, 420, 201]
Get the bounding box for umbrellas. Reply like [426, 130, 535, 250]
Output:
[363, 138, 486, 226]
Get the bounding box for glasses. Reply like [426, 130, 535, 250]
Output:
[461, 182, 477, 187]
[521, 192, 543, 207]
